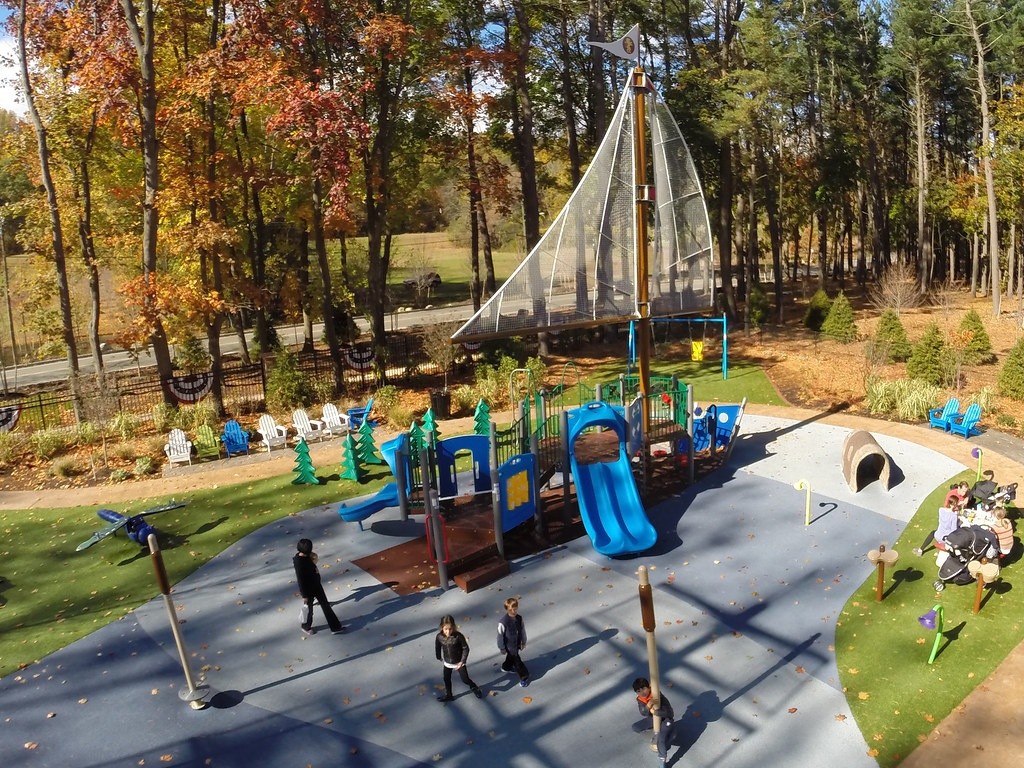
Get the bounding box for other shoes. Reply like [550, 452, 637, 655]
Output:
[500, 667, 517, 673]
[519, 677, 529, 688]
[302, 623, 314, 635]
[657, 756, 666, 768]
[437, 695, 453, 701]
[332, 626, 348, 635]
[913, 548, 922, 557]
[471, 686, 482, 698]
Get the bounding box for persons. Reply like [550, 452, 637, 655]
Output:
[496, 598, 530, 687]
[435, 615, 482, 702]
[938, 481, 1014, 559]
[293, 538, 345, 635]
[631, 678, 674, 768]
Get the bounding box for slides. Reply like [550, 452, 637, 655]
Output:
[338, 481, 402, 523]
[568, 448, 658, 558]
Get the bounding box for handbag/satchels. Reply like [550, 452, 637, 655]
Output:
[299, 604, 309, 623]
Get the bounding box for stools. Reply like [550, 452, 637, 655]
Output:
[933, 541, 951, 568]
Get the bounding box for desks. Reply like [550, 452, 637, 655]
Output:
[959, 510, 999, 536]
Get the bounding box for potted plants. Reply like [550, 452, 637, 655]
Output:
[410, 312, 464, 418]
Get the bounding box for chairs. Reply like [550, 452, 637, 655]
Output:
[194, 425, 220, 463]
[929, 399, 959, 431]
[292, 408, 325, 442]
[347, 398, 374, 430]
[321, 403, 350, 437]
[164, 428, 192, 469]
[257, 414, 288, 452]
[951, 404, 982, 439]
[222, 420, 249, 459]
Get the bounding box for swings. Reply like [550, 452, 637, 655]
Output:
[688, 321, 706, 361]
[650, 322, 669, 362]
